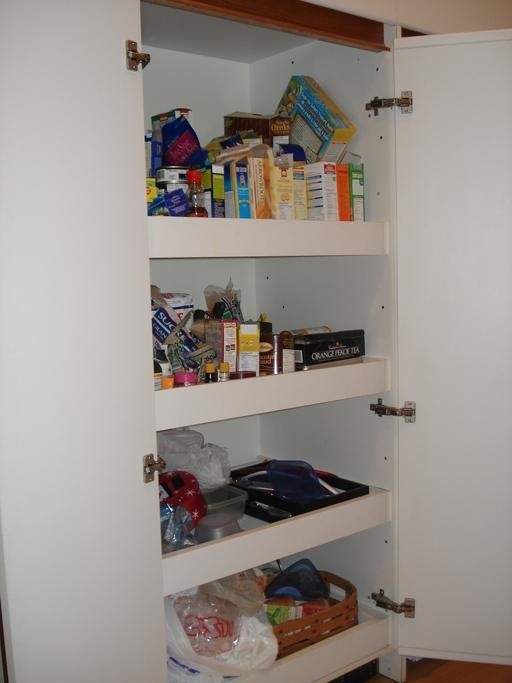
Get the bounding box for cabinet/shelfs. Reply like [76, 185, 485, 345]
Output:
[0, 1, 512, 683]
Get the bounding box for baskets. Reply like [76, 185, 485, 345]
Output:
[273, 570, 359, 660]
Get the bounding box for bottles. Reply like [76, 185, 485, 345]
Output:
[186, 170, 208, 217]
[205, 364, 214, 382]
[218, 362, 228, 380]
[174, 372, 197, 387]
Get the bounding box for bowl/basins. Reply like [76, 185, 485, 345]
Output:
[194, 514, 242, 545]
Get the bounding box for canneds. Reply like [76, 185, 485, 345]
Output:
[156, 166, 191, 201]
